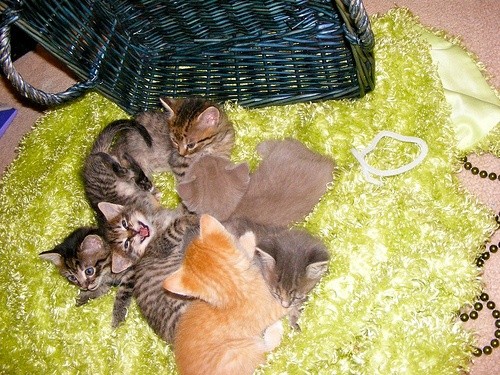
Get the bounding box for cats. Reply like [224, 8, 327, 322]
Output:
[38, 97, 337, 375]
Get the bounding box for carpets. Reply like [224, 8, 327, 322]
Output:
[0, 7, 500, 375]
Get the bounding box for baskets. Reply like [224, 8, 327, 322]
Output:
[0, 0, 375, 121]
